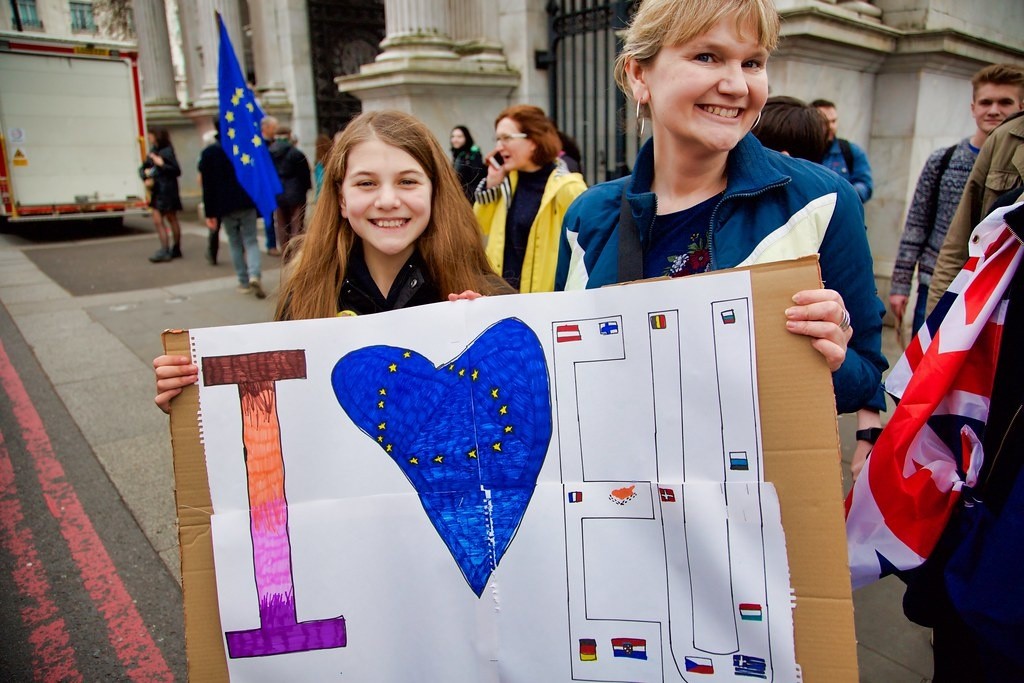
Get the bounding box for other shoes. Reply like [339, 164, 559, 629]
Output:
[170, 246, 180, 258]
[237, 285, 252, 294]
[249, 278, 266, 299]
[205, 251, 217, 265]
[266, 247, 281, 256]
[148, 247, 173, 262]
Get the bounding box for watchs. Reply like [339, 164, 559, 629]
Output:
[856, 427, 884, 443]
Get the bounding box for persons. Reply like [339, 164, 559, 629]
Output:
[751, 96, 890, 481]
[450, 118, 487, 205]
[844, 61, 1024, 682]
[559, 150, 580, 172]
[139, 128, 183, 261]
[152, 102, 519, 412]
[473, 105, 587, 294]
[555, 0, 882, 413]
[199, 113, 333, 299]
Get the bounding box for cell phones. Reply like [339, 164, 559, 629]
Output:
[485, 151, 505, 171]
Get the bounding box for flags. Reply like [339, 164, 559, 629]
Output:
[216, 11, 282, 225]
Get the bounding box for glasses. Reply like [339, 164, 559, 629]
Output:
[492, 133, 528, 145]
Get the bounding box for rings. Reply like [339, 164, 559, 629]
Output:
[838, 309, 850, 331]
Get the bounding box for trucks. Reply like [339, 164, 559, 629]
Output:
[0, 31, 150, 233]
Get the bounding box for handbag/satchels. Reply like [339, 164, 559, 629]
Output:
[900, 402, 1022, 631]
